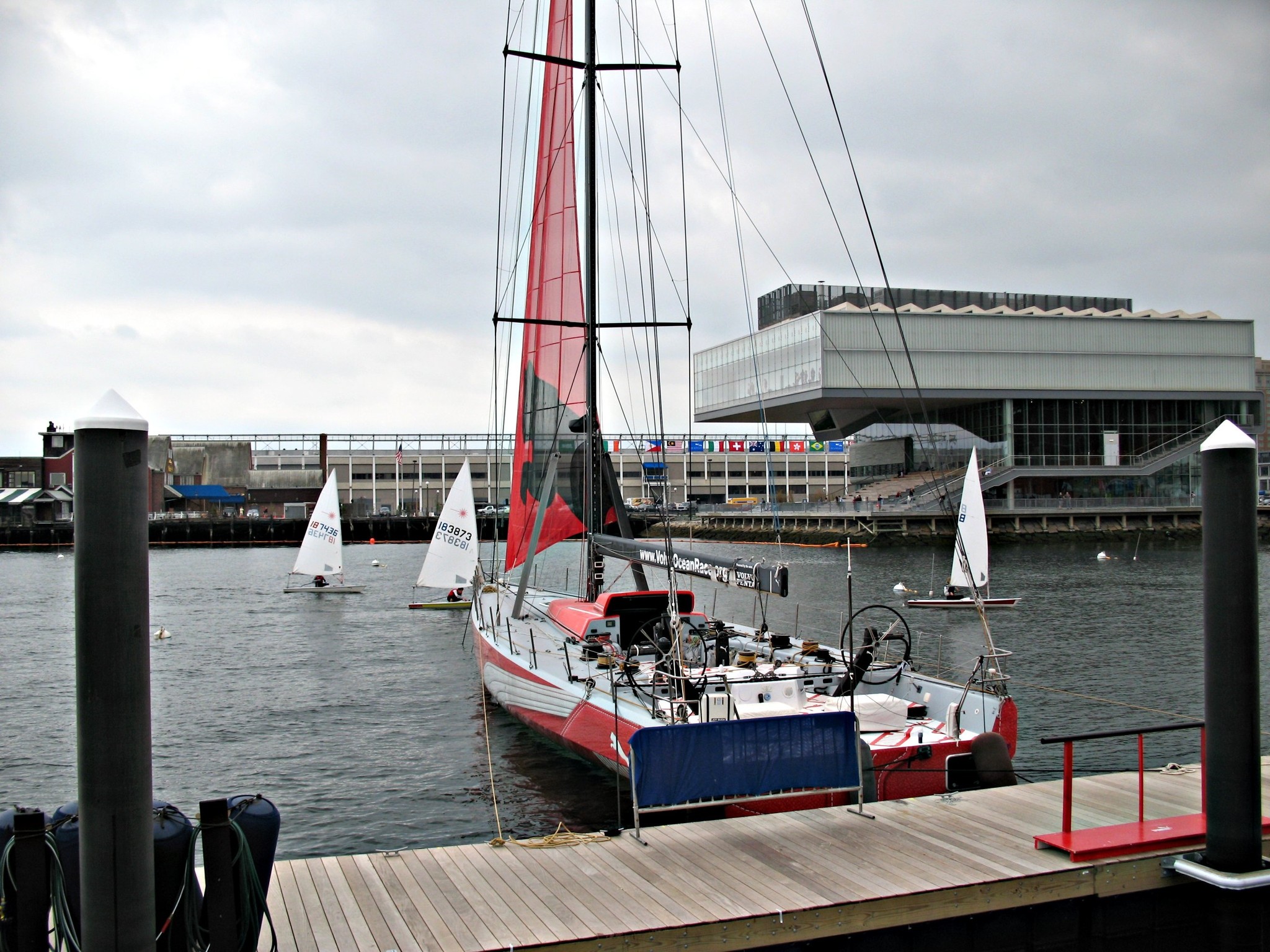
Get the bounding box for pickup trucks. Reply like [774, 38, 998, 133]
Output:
[477, 505, 498, 515]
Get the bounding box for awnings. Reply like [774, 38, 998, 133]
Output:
[643, 463, 668, 468]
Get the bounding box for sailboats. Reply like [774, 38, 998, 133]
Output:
[461, 0, 1019, 823]
[282, 469, 370, 594]
[407, 457, 487, 609]
[907, 445, 1023, 609]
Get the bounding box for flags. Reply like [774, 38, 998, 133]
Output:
[396, 444, 402, 466]
[604, 440, 843, 454]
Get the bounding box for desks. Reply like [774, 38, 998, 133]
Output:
[825, 693, 908, 731]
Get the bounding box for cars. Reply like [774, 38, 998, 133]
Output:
[624, 497, 699, 513]
[246, 509, 259, 520]
[492, 506, 510, 515]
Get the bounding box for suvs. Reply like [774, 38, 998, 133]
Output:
[222, 507, 238, 518]
[378, 507, 391, 517]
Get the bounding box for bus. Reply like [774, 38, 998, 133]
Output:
[728, 498, 758, 510]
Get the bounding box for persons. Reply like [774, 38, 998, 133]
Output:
[1057, 479, 1195, 510]
[312, 576, 329, 587]
[378, 510, 382, 518]
[877, 488, 915, 511]
[835, 496, 846, 513]
[762, 498, 766, 512]
[809, 494, 829, 502]
[263, 507, 268, 518]
[897, 463, 902, 478]
[853, 495, 862, 512]
[366, 512, 370, 517]
[984, 466, 993, 475]
[939, 488, 949, 504]
[447, 588, 468, 602]
[944, 577, 964, 600]
[5, 513, 13, 521]
[239, 507, 244, 518]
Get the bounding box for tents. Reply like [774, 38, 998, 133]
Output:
[164, 485, 244, 517]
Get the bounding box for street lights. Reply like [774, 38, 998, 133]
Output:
[436, 489, 440, 516]
[414, 489, 418, 516]
[425, 481, 430, 516]
